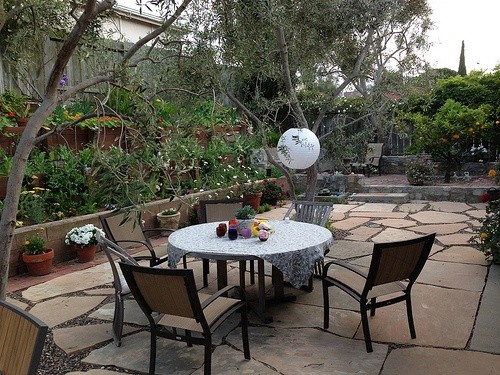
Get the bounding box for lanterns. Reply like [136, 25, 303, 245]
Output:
[276, 125, 320, 170]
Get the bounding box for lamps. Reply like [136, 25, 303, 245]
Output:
[276, 122, 321, 169]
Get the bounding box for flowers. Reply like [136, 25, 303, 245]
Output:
[406, 159, 433, 182]
[24, 226, 47, 255]
[63, 223, 106, 248]
[262, 178, 287, 200]
[466, 192, 500, 264]
[62, 109, 125, 129]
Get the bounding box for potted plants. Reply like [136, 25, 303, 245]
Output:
[234, 204, 256, 236]
[16, 102, 30, 127]
[242, 185, 262, 214]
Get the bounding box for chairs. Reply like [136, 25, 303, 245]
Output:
[98, 199, 437, 375]
[352, 143, 385, 178]
[0, 300, 49, 375]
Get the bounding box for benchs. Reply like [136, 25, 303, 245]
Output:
[250, 148, 287, 176]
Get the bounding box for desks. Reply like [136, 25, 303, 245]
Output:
[168, 219, 335, 324]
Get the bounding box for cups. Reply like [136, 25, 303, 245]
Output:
[228, 219, 238, 240]
[219, 224, 227, 232]
[216, 227, 226, 237]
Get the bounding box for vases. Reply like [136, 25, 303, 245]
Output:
[25, 248, 54, 277]
[73, 242, 100, 262]
[407, 177, 419, 185]
[0, 125, 240, 201]
[157, 209, 181, 237]
